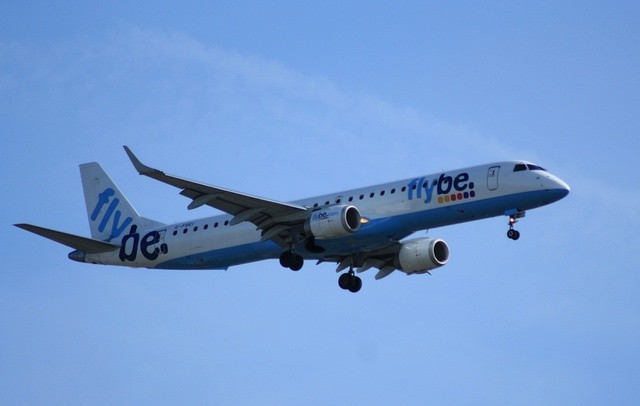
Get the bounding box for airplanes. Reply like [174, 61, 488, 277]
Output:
[12, 144, 571, 293]
